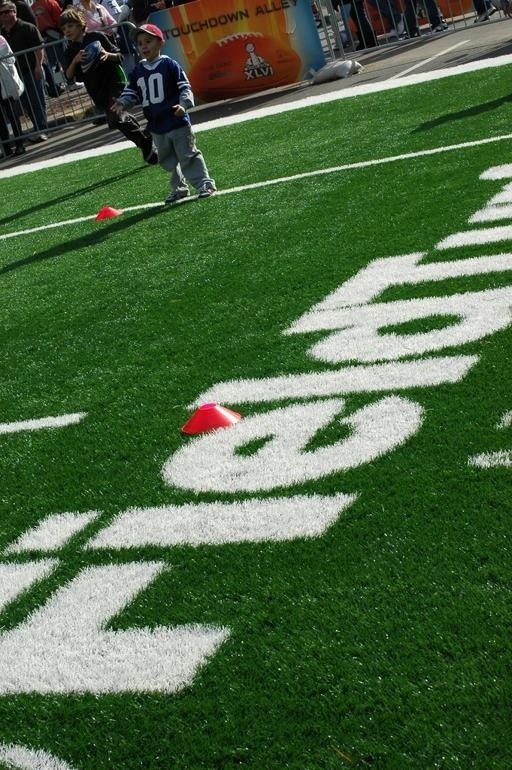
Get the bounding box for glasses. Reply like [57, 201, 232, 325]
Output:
[0, 9, 16, 14]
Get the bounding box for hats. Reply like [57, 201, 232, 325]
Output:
[130, 24, 164, 40]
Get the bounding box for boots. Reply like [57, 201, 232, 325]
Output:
[356, 30, 379, 52]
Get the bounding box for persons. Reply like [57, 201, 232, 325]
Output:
[329, 0, 512, 51]
[0, 0, 194, 166]
[109, 24, 217, 203]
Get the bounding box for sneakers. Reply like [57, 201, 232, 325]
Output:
[488, 6, 497, 17]
[0, 144, 25, 159]
[142, 135, 160, 165]
[166, 187, 189, 203]
[69, 82, 85, 91]
[198, 185, 215, 198]
[398, 30, 420, 42]
[474, 15, 490, 23]
[430, 20, 448, 34]
[36, 134, 51, 141]
